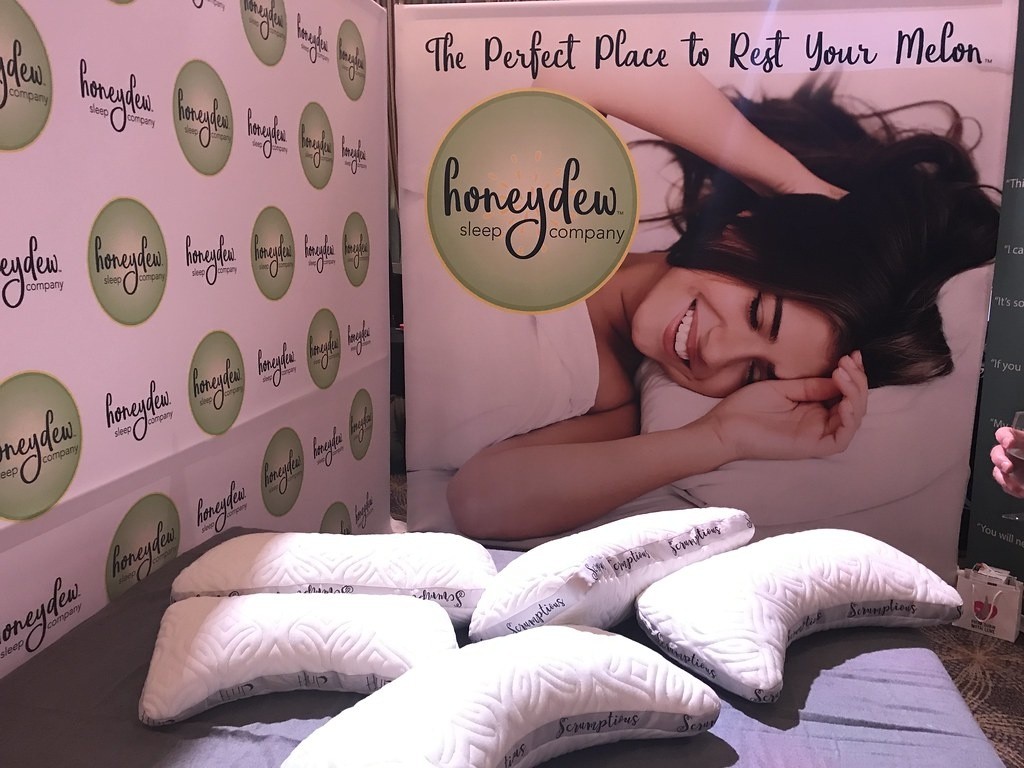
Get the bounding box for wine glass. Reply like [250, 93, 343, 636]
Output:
[1002, 411, 1024, 519]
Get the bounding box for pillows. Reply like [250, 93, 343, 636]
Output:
[633, 336, 971, 526]
[138, 591, 459, 726]
[468, 505, 755, 643]
[171, 531, 498, 628]
[634, 528, 964, 702]
[278, 624, 720, 767]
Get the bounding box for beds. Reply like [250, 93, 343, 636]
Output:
[0, 525, 1010, 766]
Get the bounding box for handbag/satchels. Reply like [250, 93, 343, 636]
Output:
[951, 563, 1024, 643]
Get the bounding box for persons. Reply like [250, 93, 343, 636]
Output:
[397, 41, 1000, 539]
